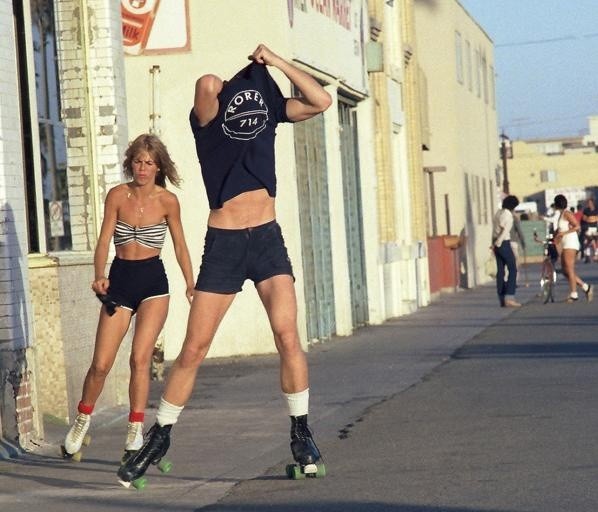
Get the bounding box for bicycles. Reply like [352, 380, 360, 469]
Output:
[534, 233, 563, 304]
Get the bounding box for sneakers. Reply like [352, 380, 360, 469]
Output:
[505, 298, 521, 306]
[585, 284, 593, 303]
[565, 296, 578, 302]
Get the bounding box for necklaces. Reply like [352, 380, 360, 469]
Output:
[123, 187, 148, 223]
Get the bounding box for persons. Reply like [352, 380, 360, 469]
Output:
[552, 195, 595, 304]
[113, 42, 337, 480]
[520, 198, 597, 264]
[491, 194, 523, 308]
[60, 132, 197, 455]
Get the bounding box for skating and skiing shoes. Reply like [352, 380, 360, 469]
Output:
[121, 422, 145, 464]
[116, 420, 173, 489]
[286, 415, 327, 480]
[59, 413, 91, 461]
